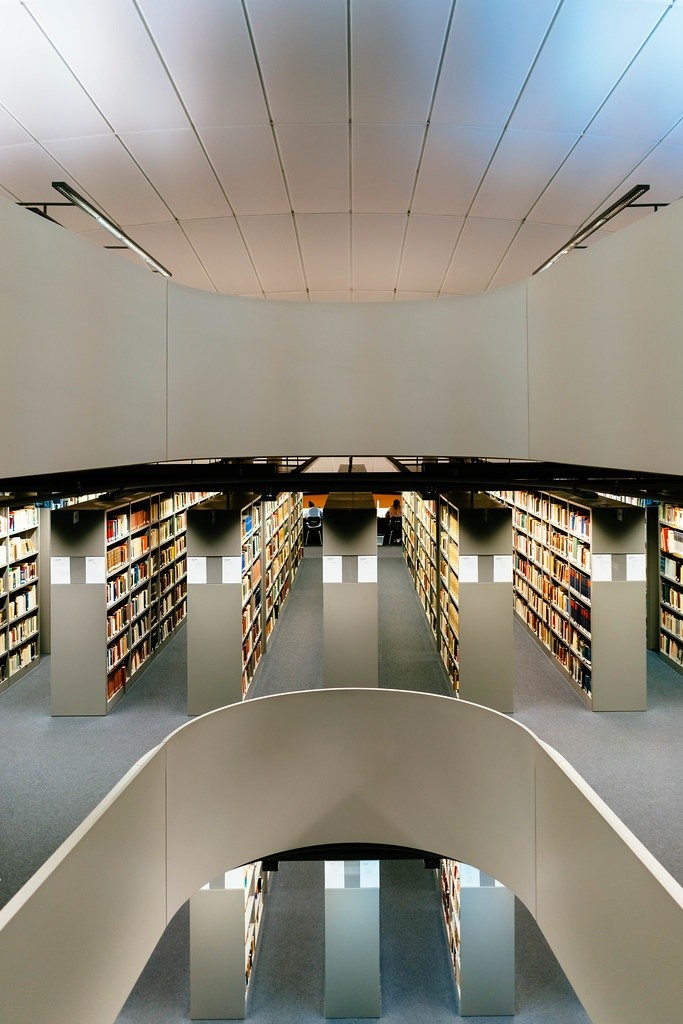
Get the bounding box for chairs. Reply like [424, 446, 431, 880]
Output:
[388, 516, 402, 545]
[305, 517, 323, 546]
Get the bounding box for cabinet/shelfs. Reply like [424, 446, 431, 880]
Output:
[323, 860, 381, 1018]
[434, 856, 516, 1017]
[190, 861, 272, 1020]
[401, 490, 683, 712]
[0, 491, 304, 717]
[323, 465, 379, 688]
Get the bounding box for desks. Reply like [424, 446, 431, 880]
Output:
[302, 507, 391, 546]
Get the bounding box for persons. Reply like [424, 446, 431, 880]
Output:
[386, 499, 402, 543]
[306, 500, 323, 545]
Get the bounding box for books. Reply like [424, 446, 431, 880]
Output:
[244, 861, 263, 1007]
[401, 488, 460, 699]
[106, 492, 224, 699]
[484, 490, 592, 697]
[0, 490, 105, 684]
[436, 856, 461, 1006]
[242, 491, 304, 700]
[595, 492, 683, 666]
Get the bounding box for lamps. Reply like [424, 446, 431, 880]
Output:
[13, 182, 173, 277]
[532, 184, 671, 277]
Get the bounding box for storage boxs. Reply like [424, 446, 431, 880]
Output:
[377, 533, 385, 545]
